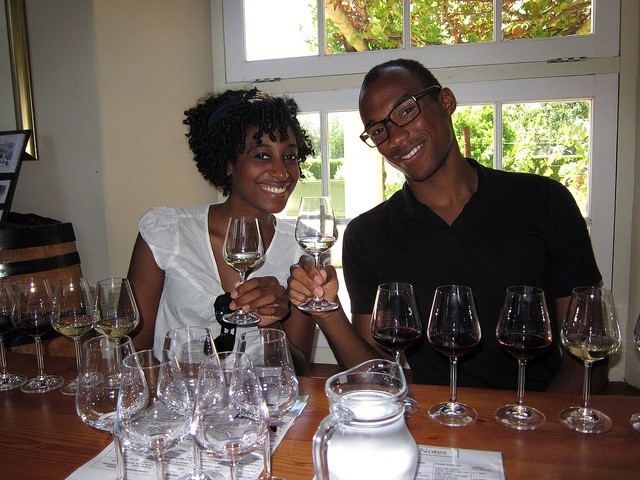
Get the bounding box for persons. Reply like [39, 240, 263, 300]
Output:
[287, 59, 609, 395]
[118, 87, 331, 376]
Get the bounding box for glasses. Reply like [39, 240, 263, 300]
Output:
[213, 292, 236, 360]
[359, 85, 442, 147]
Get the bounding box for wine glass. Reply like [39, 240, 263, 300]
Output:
[74, 334, 149, 480]
[229, 328, 299, 480]
[426, 285, 481, 427]
[369, 282, 422, 418]
[10, 277, 64, 393]
[115, 349, 192, 480]
[91, 278, 141, 390]
[495, 286, 553, 430]
[629, 314, 640, 432]
[51, 277, 106, 397]
[191, 351, 270, 480]
[156, 326, 226, 480]
[0, 280, 27, 392]
[558, 286, 621, 434]
[222, 216, 264, 325]
[295, 197, 339, 311]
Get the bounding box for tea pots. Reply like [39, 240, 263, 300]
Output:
[312, 358, 419, 480]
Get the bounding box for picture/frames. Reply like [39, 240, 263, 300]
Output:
[1, 0, 39, 163]
